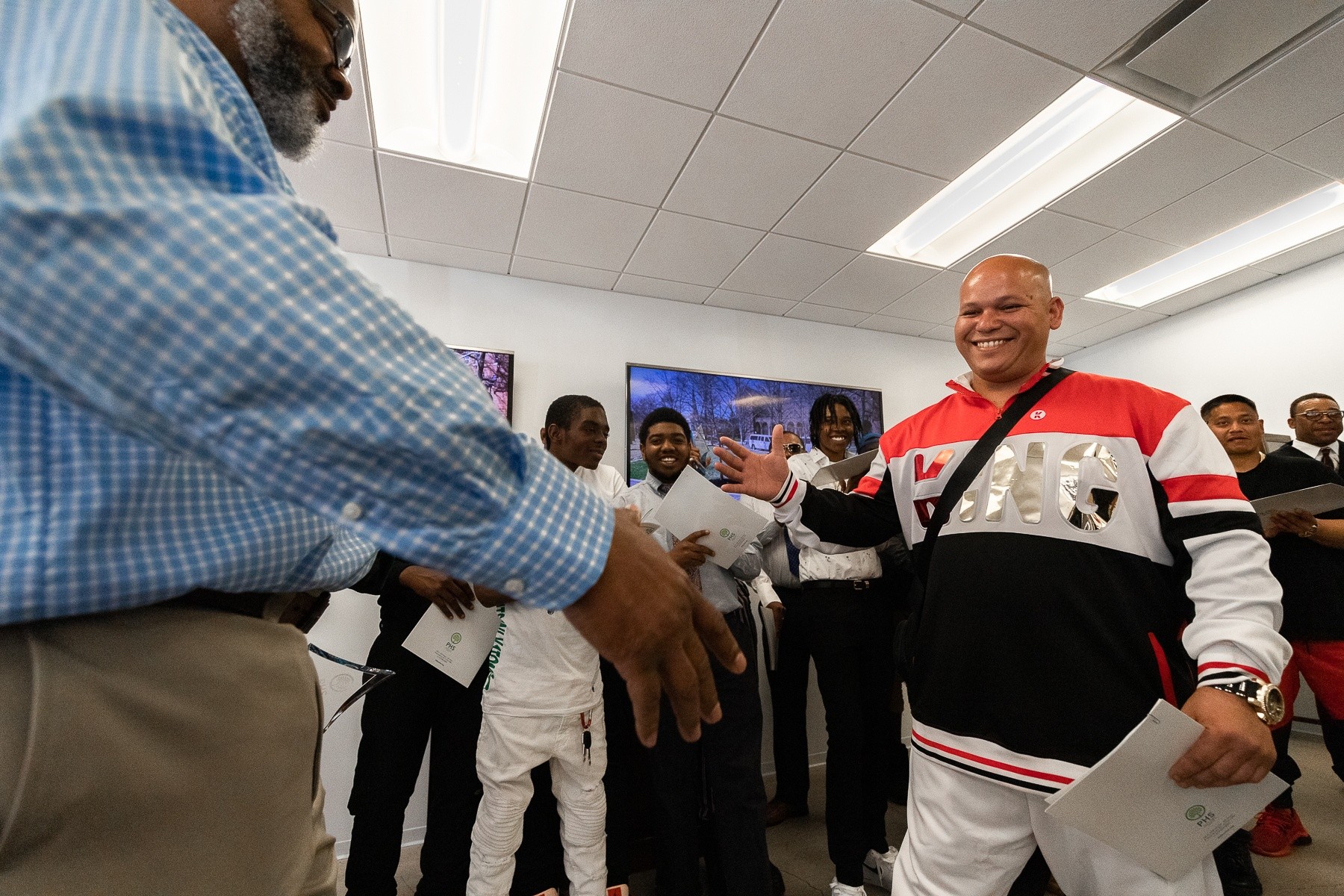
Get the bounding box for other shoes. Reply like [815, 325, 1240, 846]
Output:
[766, 797, 809, 825]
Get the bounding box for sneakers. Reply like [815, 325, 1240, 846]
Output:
[1245, 807, 1312, 859]
[829, 876, 867, 896]
[862, 845, 898, 890]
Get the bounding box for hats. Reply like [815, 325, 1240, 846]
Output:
[855, 432, 883, 455]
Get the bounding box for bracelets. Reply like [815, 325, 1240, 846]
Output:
[1298, 525, 1318, 538]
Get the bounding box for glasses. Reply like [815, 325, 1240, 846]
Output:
[783, 443, 804, 454]
[318, 0, 356, 77]
[1295, 408, 1344, 421]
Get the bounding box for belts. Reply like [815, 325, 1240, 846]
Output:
[196, 586, 331, 634]
[798, 579, 874, 596]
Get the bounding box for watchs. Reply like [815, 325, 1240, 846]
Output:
[1210, 680, 1284, 725]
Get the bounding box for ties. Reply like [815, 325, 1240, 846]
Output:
[1321, 449, 1335, 471]
[784, 524, 800, 577]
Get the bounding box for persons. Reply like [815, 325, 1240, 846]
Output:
[0, 0, 748, 896]
[345, 393, 910, 896]
[1171, 393, 1344, 896]
[713, 253, 1293, 896]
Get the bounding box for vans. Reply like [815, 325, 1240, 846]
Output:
[748, 434, 772, 451]
